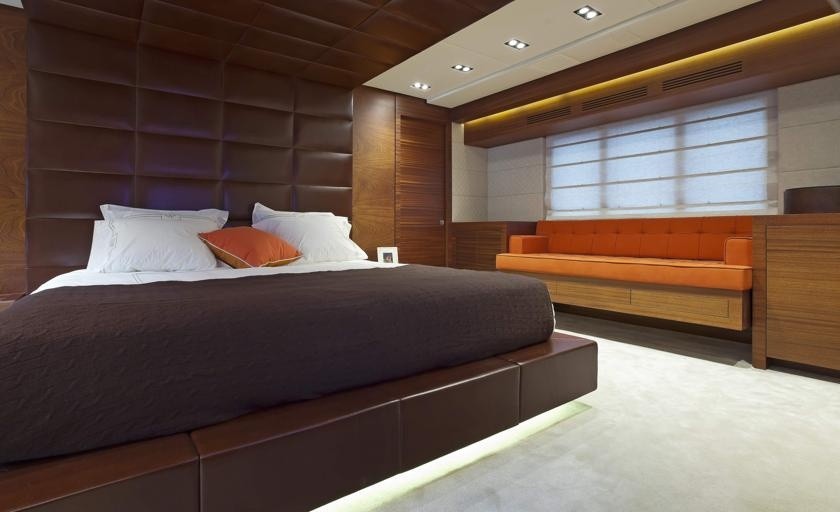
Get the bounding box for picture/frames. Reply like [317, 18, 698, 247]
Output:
[377, 246, 399, 264]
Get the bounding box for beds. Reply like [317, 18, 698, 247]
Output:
[0, 259, 597, 512]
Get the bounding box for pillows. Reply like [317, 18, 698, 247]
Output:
[84, 220, 217, 271]
[252, 215, 369, 262]
[251, 201, 353, 239]
[103, 205, 231, 228]
[198, 225, 303, 268]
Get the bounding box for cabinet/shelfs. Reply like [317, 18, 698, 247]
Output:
[750, 214, 840, 371]
[450, 221, 536, 271]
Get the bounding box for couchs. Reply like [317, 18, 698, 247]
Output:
[494, 213, 755, 343]
